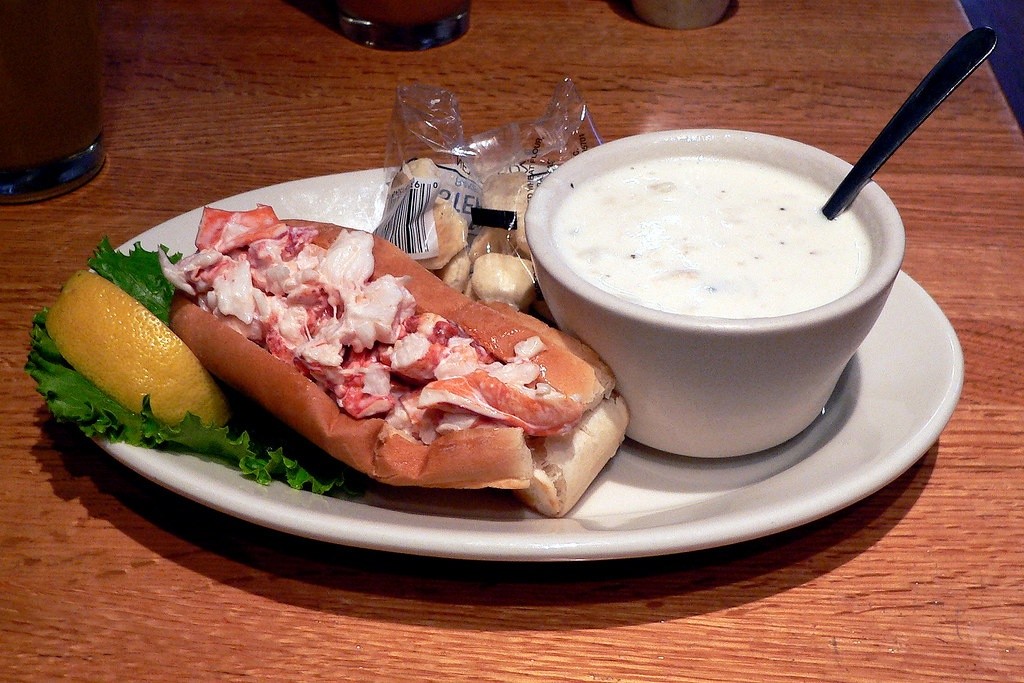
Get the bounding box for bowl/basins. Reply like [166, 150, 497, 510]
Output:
[524, 128, 906, 457]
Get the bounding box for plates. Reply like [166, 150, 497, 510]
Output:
[86, 169, 962, 559]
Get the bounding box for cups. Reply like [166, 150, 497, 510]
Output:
[337, 0, 473, 52]
[632, 0, 730, 29]
[0, 0, 107, 204]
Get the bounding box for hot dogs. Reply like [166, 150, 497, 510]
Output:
[166, 210, 628, 514]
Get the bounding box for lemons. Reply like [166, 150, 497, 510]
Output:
[45, 269, 230, 433]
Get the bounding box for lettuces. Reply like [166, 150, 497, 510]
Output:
[23, 232, 372, 498]
